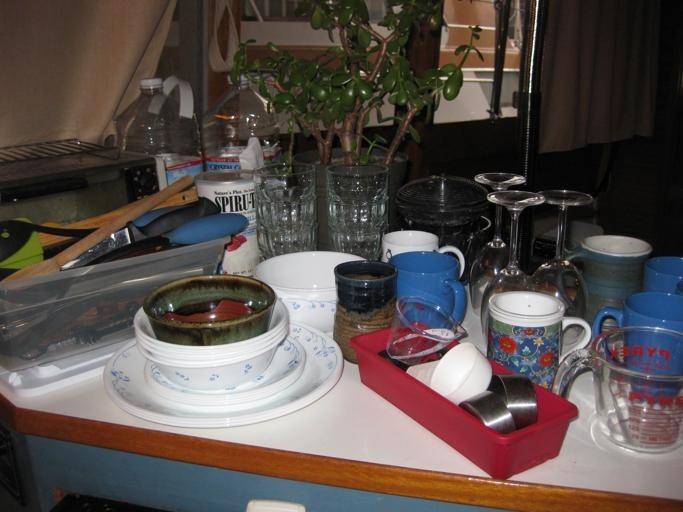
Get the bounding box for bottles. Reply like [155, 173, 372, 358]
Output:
[113, 68, 283, 189]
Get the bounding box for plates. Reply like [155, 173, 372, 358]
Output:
[105, 320, 342, 431]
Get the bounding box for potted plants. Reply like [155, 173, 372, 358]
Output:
[225, 0, 489, 262]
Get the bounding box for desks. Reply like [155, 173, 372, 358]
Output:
[0, 284, 683, 511]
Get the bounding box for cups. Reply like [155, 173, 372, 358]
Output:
[253, 161, 318, 255]
[486, 290, 592, 391]
[551, 231, 683, 455]
[326, 163, 390, 262]
[404, 341, 541, 436]
[333, 230, 467, 367]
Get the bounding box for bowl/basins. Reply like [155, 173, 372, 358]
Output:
[127, 275, 295, 389]
[259, 248, 367, 333]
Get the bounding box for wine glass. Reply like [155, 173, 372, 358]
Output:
[470, 171, 591, 318]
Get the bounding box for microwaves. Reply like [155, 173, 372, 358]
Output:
[0, 139, 159, 231]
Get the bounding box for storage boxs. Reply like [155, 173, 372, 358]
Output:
[345, 321, 580, 482]
[1, 231, 234, 365]
